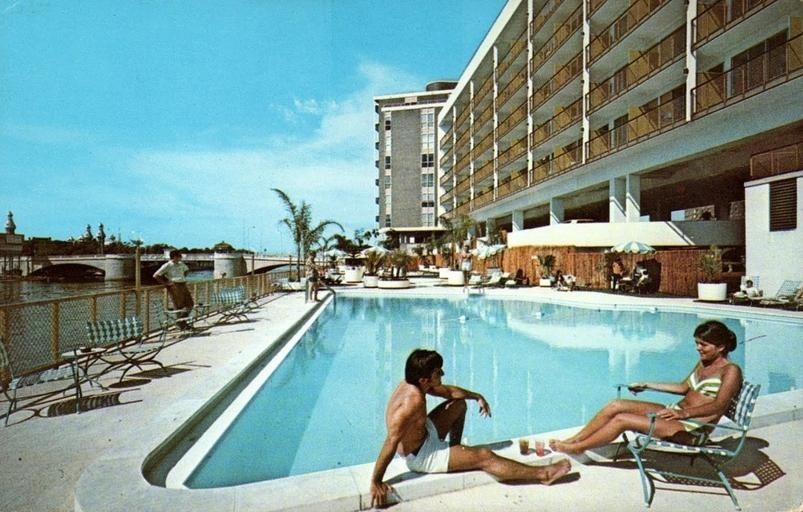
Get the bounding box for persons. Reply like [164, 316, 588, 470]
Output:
[306, 251, 321, 301]
[370, 349, 571, 507]
[461, 245, 474, 287]
[733, 280, 760, 298]
[549, 319, 743, 455]
[612, 257, 649, 292]
[556, 270, 564, 290]
[153, 250, 194, 330]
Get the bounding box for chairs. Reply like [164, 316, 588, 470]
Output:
[0, 300, 201, 430]
[611, 375, 762, 511]
[732, 275, 803, 311]
[210, 285, 263, 324]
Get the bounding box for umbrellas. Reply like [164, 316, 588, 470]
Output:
[605, 240, 656, 276]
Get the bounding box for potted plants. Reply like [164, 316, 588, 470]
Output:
[533, 254, 556, 287]
[429, 214, 506, 284]
[342, 238, 413, 287]
[697, 242, 727, 301]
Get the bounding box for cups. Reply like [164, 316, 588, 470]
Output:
[535, 440, 545, 456]
[520, 438, 529, 456]
